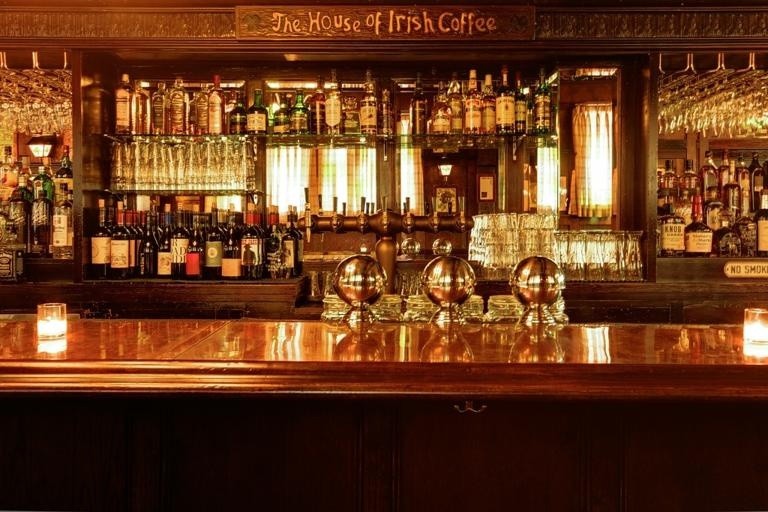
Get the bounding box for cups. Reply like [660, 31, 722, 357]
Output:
[743, 308, 768, 341]
[472, 213, 646, 286]
[742, 341, 768, 365]
[37, 302, 67, 339]
[309, 272, 566, 325]
[37, 340, 66, 360]
[110, 136, 257, 191]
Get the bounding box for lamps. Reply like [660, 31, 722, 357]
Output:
[27, 136, 56, 158]
[436, 159, 457, 184]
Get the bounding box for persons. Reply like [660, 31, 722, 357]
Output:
[243, 244, 256, 280]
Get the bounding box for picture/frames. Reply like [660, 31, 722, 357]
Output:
[478, 175, 496, 202]
[433, 186, 458, 215]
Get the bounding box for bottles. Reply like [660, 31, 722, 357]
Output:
[656, 152, 767, 256]
[90, 194, 302, 284]
[69, 321, 243, 359]
[428, 67, 554, 132]
[653, 328, 743, 367]
[0, 144, 79, 283]
[0, 318, 36, 359]
[115, 69, 396, 134]
[409, 72, 427, 135]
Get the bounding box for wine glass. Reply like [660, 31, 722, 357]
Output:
[657, 52, 768, 138]
[0, 48, 75, 139]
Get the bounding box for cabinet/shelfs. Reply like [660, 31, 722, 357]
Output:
[108, 76, 556, 213]
